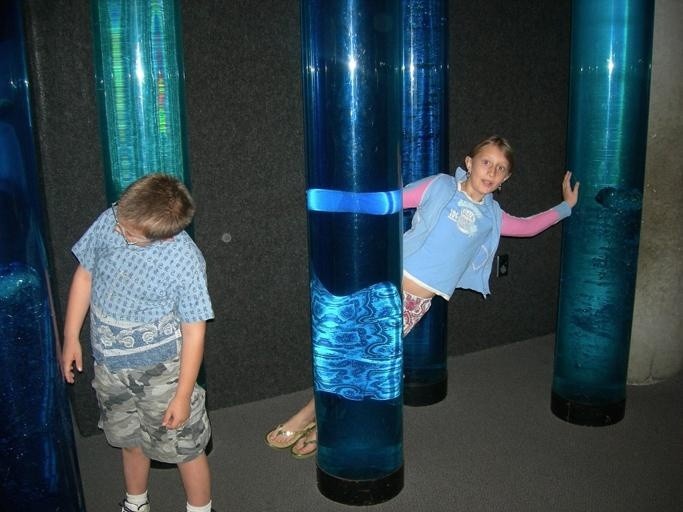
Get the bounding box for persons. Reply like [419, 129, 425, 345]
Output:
[60, 172, 215, 512]
[265, 134, 582, 460]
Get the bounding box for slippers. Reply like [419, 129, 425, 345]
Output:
[291, 426, 317, 460]
[264, 415, 317, 450]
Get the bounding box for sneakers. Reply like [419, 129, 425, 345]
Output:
[119, 499, 150, 512]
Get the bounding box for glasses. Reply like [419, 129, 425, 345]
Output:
[110, 201, 159, 247]
